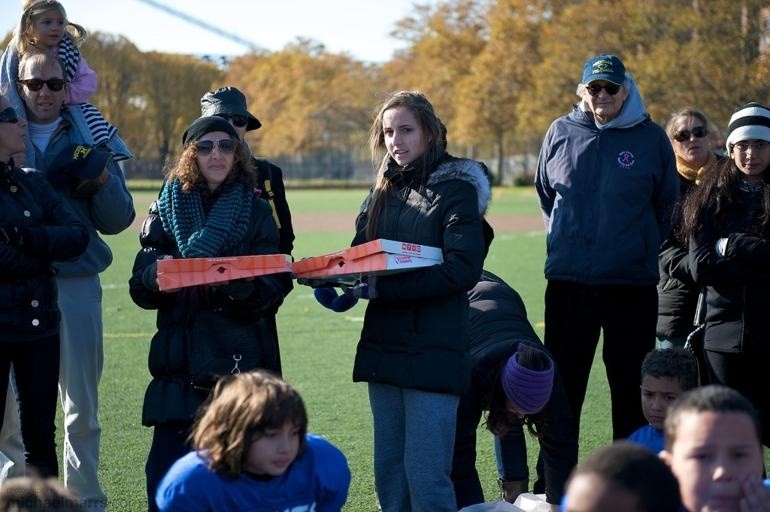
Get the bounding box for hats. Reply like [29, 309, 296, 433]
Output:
[581, 53, 627, 86]
[200, 85, 262, 131]
[182, 116, 239, 148]
[726, 101, 770, 154]
[47, 143, 129, 187]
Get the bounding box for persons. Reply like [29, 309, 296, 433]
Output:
[657, 384, 770, 512]
[564, 440, 681, 512]
[534, 53, 683, 502]
[128, 115, 294, 512]
[0, 0, 98, 194]
[154, 369, 352, 512]
[199, 86, 295, 294]
[0, 477, 86, 511]
[0, 48, 135, 511]
[627, 347, 696, 454]
[656, 110, 725, 348]
[0, 95, 91, 479]
[296, 89, 494, 512]
[450, 270, 556, 510]
[684, 101, 770, 438]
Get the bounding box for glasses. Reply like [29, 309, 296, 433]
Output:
[733, 143, 770, 151]
[16, 77, 67, 92]
[586, 83, 624, 96]
[0, 106, 20, 126]
[216, 113, 249, 128]
[673, 126, 709, 142]
[193, 138, 236, 153]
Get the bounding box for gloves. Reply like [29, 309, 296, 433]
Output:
[342, 275, 396, 301]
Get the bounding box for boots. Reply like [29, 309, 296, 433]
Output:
[493, 469, 531, 505]
